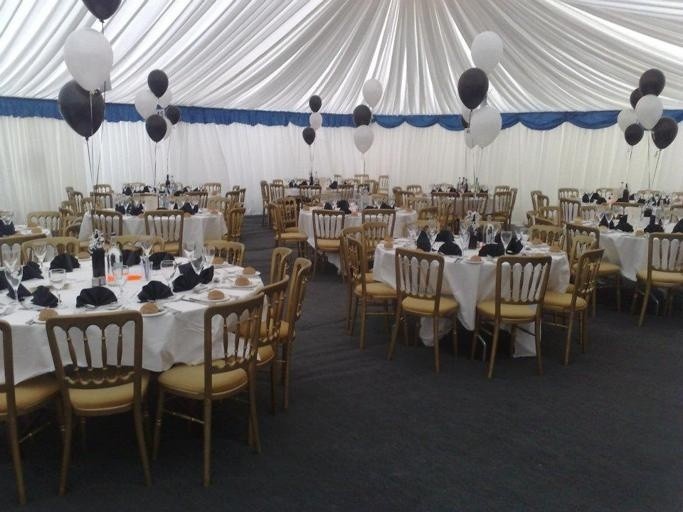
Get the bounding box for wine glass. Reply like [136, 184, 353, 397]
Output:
[113, 265, 129, 297]
[346, 194, 395, 216]
[644, 191, 673, 209]
[161, 260, 176, 286]
[205, 246, 216, 266]
[140, 232, 153, 256]
[595, 210, 604, 227]
[5, 266, 22, 306]
[405, 223, 418, 246]
[605, 212, 613, 231]
[1, 247, 20, 268]
[183, 239, 197, 258]
[50, 269, 66, 305]
[33, 240, 46, 268]
[500, 230, 513, 259]
[190, 250, 204, 276]
[424, 217, 439, 253]
[429, 183, 453, 192]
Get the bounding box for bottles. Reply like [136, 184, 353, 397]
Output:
[469, 215, 478, 249]
[619, 181, 625, 198]
[107, 231, 124, 281]
[309, 172, 313, 185]
[86, 231, 105, 286]
[623, 184, 629, 202]
[484, 215, 495, 245]
[457, 176, 480, 193]
[313, 170, 319, 185]
[166, 175, 170, 188]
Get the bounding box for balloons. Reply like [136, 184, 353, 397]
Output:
[55, 78, 105, 142]
[352, 104, 371, 127]
[456, 67, 490, 111]
[63, 28, 112, 95]
[467, 106, 504, 149]
[471, 31, 504, 75]
[352, 124, 374, 155]
[308, 95, 323, 114]
[308, 112, 323, 132]
[463, 130, 476, 150]
[362, 78, 383, 108]
[82, 1, 122, 21]
[301, 126, 316, 147]
[617, 68, 678, 151]
[136, 68, 179, 143]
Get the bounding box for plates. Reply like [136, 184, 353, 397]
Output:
[33, 313, 60, 324]
[200, 293, 231, 302]
[232, 281, 258, 288]
[138, 308, 168, 317]
[466, 259, 484, 264]
[237, 269, 262, 276]
